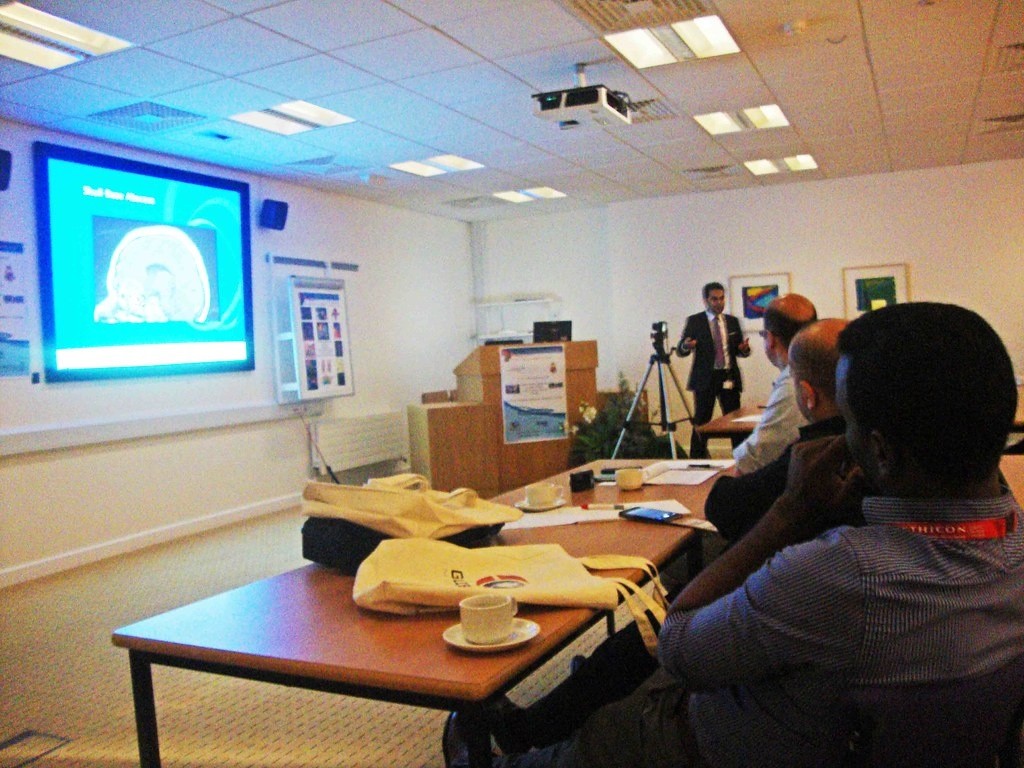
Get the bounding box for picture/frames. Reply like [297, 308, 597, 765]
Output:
[729, 275, 790, 331]
[842, 264, 912, 321]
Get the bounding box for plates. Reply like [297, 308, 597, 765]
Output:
[515, 498, 568, 511]
[442, 618, 540, 652]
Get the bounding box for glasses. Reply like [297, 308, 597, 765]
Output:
[758, 331, 778, 339]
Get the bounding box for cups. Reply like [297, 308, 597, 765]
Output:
[526, 481, 564, 505]
[459, 593, 518, 645]
[615, 469, 650, 491]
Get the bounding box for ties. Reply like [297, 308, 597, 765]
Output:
[713, 317, 725, 369]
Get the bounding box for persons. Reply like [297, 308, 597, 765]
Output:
[485, 316, 846, 749]
[445, 302, 1024, 768]
[677, 283, 750, 459]
[731, 294, 817, 474]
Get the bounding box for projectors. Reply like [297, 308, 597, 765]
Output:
[530, 84, 633, 130]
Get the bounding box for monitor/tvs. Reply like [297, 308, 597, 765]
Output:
[533, 321, 572, 343]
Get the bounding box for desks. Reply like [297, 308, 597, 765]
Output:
[113, 459, 737, 768]
[698, 399, 764, 447]
[408, 341, 648, 499]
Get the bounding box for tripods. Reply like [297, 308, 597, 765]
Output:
[612, 332, 712, 459]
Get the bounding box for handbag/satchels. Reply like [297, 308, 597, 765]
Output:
[300, 472, 525, 542]
[352, 537, 670, 658]
[301, 516, 504, 577]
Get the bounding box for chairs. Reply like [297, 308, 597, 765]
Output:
[421, 391, 448, 401]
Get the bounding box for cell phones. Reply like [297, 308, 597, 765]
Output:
[620, 506, 682, 522]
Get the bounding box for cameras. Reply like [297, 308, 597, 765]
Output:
[652, 322, 667, 333]
[570, 470, 594, 492]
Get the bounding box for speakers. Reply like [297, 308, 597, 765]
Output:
[260, 199, 289, 230]
[0, 148, 11, 190]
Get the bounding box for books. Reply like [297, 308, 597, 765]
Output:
[644, 463, 719, 484]
